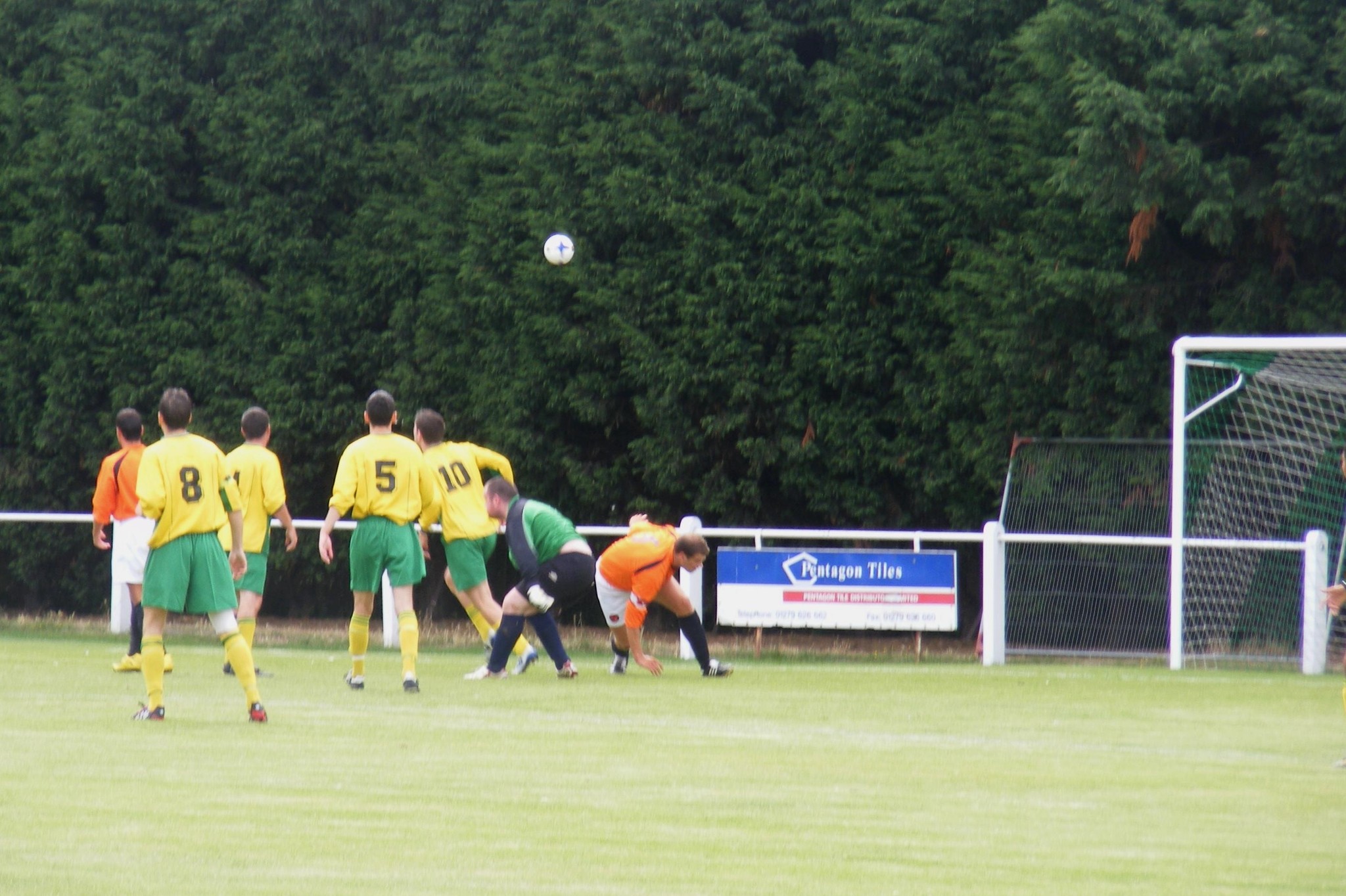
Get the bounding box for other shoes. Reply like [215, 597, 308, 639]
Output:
[344, 671, 364, 689]
[403, 678, 418, 694]
[224, 661, 258, 675]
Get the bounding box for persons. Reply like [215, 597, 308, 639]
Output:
[321, 391, 436, 692]
[408, 407, 539, 676]
[220, 407, 297, 674]
[597, 514, 733, 676]
[466, 478, 599, 680]
[92, 409, 177, 673]
[132, 387, 265, 723]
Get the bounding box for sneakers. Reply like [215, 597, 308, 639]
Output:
[248, 702, 268, 722]
[163, 653, 173, 672]
[481, 628, 497, 662]
[132, 703, 164, 720]
[112, 652, 144, 672]
[556, 659, 578, 679]
[512, 644, 538, 674]
[464, 665, 507, 679]
[701, 660, 732, 677]
[610, 652, 628, 674]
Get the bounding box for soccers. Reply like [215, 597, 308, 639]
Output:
[544, 234, 574, 266]
